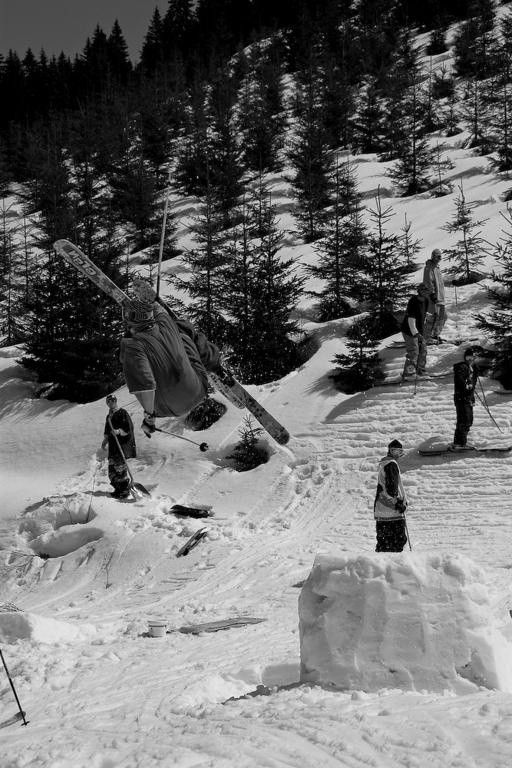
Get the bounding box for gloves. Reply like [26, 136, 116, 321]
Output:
[416, 332, 425, 342]
[140, 410, 156, 439]
[429, 293, 438, 303]
[394, 499, 406, 514]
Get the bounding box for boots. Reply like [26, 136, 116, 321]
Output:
[109, 478, 129, 499]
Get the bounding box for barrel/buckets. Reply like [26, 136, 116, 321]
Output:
[148, 620, 166, 637]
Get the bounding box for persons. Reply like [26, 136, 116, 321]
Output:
[424, 249, 447, 345]
[399, 284, 434, 381]
[373, 439, 409, 552]
[101, 394, 136, 499]
[452, 348, 480, 451]
[121, 293, 222, 439]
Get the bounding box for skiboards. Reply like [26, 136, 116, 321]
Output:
[53, 239, 290, 446]
[386, 338, 479, 349]
[373, 370, 454, 387]
[417, 446, 512, 456]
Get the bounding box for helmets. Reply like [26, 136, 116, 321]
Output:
[416, 283, 428, 292]
[431, 248, 442, 257]
[464, 349, 473, 356]
[105, 394, 116, 402]
[121, 296, 153, 328]
[388, 439, 402, 449]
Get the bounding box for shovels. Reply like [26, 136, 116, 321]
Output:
[108, 417, 151, 502]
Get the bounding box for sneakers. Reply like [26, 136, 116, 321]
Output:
[424, 336, 440, 344]
[400, 371, 431, 379]
[447, 442, 476, 451]
